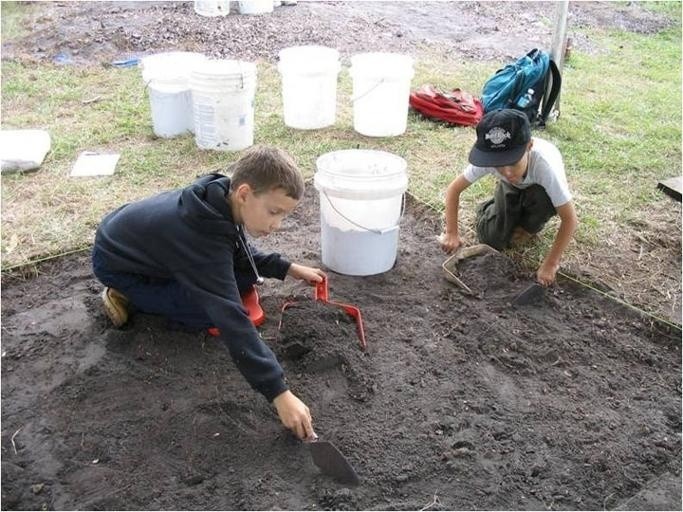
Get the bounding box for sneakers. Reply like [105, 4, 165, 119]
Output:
[508, 226, 535, 248]
[102, 286, 129, 329]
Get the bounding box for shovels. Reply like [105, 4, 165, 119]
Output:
[513, 283, 543, 305]
[308, 434, 363, 487]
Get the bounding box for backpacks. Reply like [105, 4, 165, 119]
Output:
[409, 83, 485, 129]
[480, 48, 561, 131]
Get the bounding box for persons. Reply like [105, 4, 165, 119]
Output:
[91, 143, 330, 445]
[435, 108, 578, 288]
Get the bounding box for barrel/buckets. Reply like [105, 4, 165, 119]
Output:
[348, 52, 414, 138]
[276, 44, 341, 131]
[187, 59, 257, 154]
[140, 50, 206, 139]
[237, 1, 275, 15]
[192, 1, 232, 18]
[313, 149, 409, 277]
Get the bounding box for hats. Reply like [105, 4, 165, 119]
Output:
[468, 107, 532, 168]
